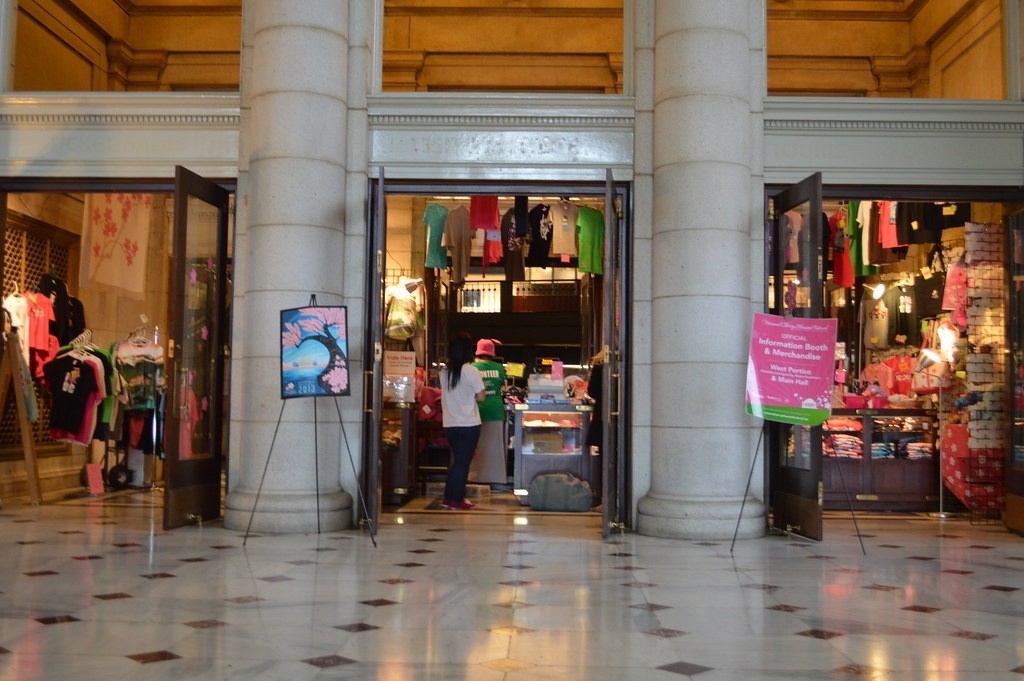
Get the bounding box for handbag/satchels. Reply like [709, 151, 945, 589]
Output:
[529, 474, 592, 511]
[910, 321, 951, 394]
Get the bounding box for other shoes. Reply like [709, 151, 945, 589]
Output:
[492, 484, 508, 489]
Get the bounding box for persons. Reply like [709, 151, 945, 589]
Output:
[584, 347, 616, 506]
[439, 330, 515, 510]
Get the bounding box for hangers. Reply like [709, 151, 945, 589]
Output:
[28, 280, 39, 294]
[130, 326, 150, 344]
[896, 271, 911, 287]
[541, 196, 597, 211]
[49, 264, 61, 280]
[839, 200, 848, 213]
[11, 280, 22, 297]
[57, 329, 99, 365]
[181, 368, 190, 389]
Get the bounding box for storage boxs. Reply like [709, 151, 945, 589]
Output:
[842, 395, 863, 408]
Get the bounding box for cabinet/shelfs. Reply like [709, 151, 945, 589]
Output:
[514, 404, 597, 506]
[788, 409, 963, 513]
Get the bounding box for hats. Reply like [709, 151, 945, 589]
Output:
[475, 338, 495, 356]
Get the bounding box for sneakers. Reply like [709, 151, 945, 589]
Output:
[440, 498, 450, 507]
[449, 500, 476, 509]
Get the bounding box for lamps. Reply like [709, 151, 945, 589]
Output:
[915, 348, 958, 518]
[859, 283, 885, 382]
[405, 278, 428, 386]
